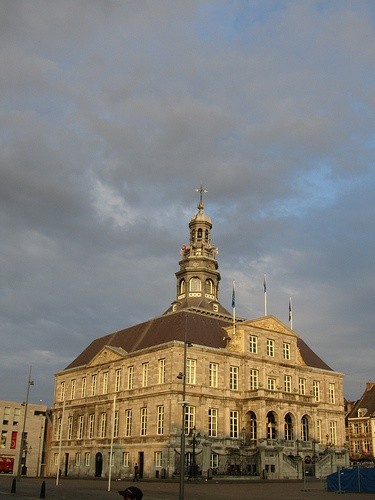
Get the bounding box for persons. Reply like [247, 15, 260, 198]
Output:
[118, 486, 143, 500]
[133, 463, 139, 482]
[264, 468, 270, 481]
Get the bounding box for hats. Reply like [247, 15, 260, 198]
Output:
[119, 486, 143, 500]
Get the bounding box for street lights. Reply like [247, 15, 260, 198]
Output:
[16, 364, 35, 482]
[177, 336, 194, 500]
